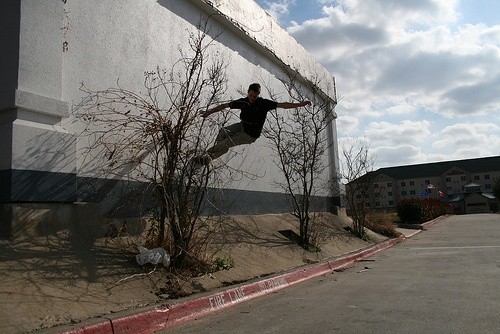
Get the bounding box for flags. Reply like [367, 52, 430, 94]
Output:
[427, 184, 435, 189]
[419, 191, 428, 196]
[438, 191, 444, 196]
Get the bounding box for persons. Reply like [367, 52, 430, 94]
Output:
[197, 82, 311, 166]
